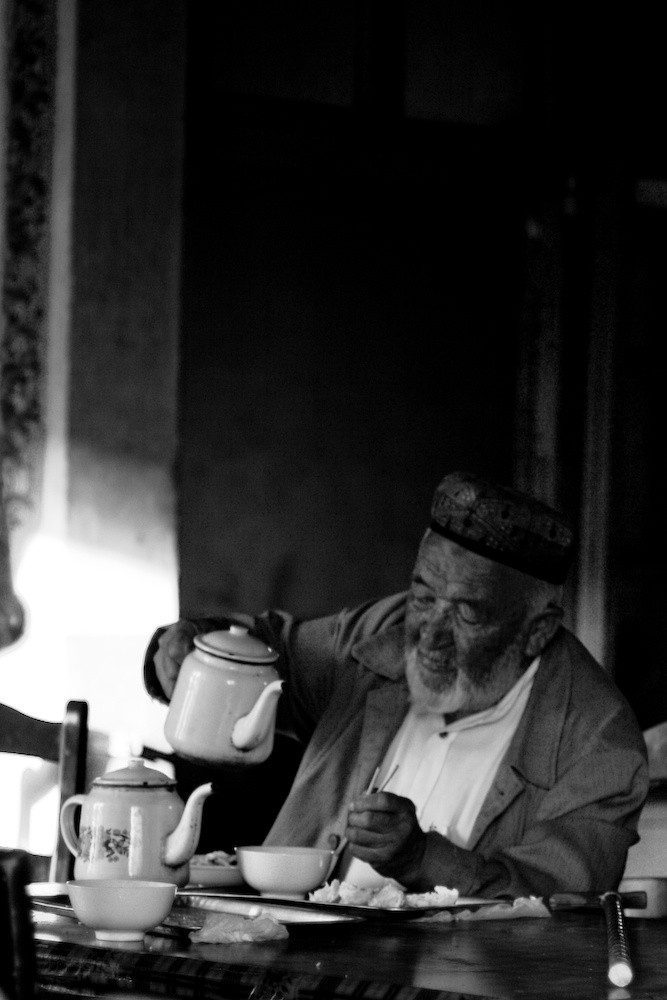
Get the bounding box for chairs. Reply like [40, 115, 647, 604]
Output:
[0, 700, 90, 880]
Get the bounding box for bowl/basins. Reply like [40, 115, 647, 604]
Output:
[189, 865, 243, 887]
[236, 846, 331, 900]
[65, 879, 177, 941]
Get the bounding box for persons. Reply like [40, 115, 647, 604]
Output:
[143, 468, 651, 1000]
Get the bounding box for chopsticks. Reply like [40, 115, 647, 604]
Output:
[335, 765, 399, 855]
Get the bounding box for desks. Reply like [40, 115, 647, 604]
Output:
[37, 910, 667, 1000]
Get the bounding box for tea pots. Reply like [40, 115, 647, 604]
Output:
[163, 625, 285, 764]
[60, 760, 213, 887]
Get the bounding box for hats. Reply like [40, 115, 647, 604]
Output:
[430, 471, 578, 585]
